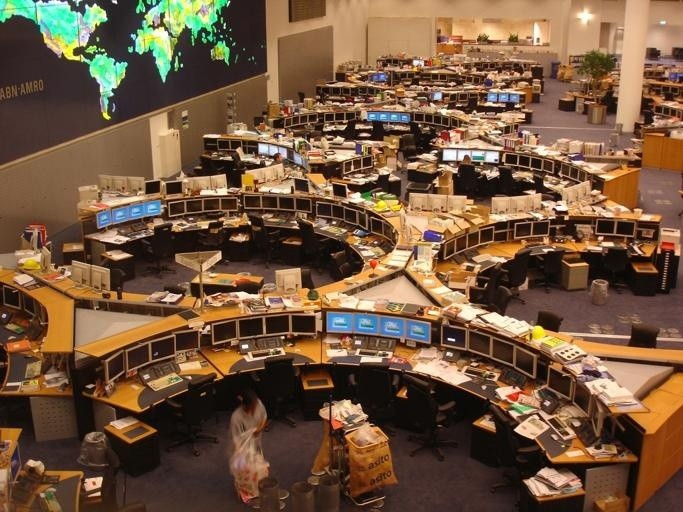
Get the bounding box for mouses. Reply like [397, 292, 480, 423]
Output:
[550, 433, 560, 440]
[8, 335, 16, 340]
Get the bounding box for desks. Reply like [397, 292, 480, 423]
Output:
[1, 65, 681, 510]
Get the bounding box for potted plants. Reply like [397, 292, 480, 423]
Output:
[576, 47, 617, 126]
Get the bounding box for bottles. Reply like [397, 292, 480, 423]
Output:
[116, 286, 123, 300]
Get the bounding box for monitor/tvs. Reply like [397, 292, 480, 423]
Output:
[294, 177, 310, 192]
[172, 329, 200, 355]
[72, 260, 111, 291]
[145, 180, 160, 194]
[442, 149, 499, 165]
[2, 286, 46, 326]
[104, 350, 125, 383]
[414, 113, 460, 129]
[504, 153, 555, 174]
[441, 324, 538, 379]
[284, 114, 318, 128]
[168, 197, 238, 217]
[342, 155, 373, 174]
[561, 163, 591, 182]
[332, 183, 348, 198]
[243, 194, 312, 215]
[95, 199, 161, 229]
[562, 180, 591, 204]
[314, 58, 529, 104]
[211, 313, 317, 348]
[324, 112, 356, 124]
[661, 85, 679, 95]
[643, 71, 662, 77]
[125, 342, 150, 372]
[9, 445, 22, 486]
[514, 220, 549, 239]
[491, 192, 541, 213]
[326, 310, 431, 345]
[547, 366, 605, 437]
[370, 216, 398, 246]
[409, 193, 467, 213]
[444, 226, 494, 260]
[315, 201, 368, 230]
[367, 112, 411, 125]
[654, 105, 683, 120]
[595, 218, 636, 241]
[165, 181, 183, 194]
[150, 335, 176, 363]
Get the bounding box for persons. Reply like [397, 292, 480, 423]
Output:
[229, 380, 268, 509]
[270, 153, 283, 166]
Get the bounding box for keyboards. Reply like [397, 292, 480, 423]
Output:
[12, 473, 46, 504]
[138, 361, 181, 385]
[464, 366, 496, 379]
[0, 310, 14, 325]
[352, 336, 396, 352]
[548, 415, 577, 440]
[239, 337, 283, 354]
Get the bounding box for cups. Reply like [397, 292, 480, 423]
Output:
[634, 208, 643, 218]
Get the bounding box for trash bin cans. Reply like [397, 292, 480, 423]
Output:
[80, 431, 112, 469]
[608, 131, 619, 146]
[311, 399, 399, 498]
[590, 279, 609, 305]
[406, 182, 432, 193]
